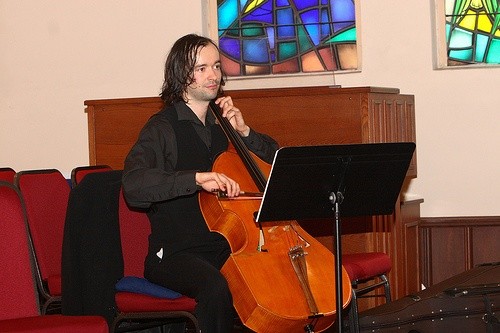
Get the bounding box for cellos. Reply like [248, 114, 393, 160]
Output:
[199, 92, 352, 333]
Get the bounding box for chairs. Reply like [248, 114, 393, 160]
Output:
[0, 164, 393, 333]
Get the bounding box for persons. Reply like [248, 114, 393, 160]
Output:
[122, 34, 280, 333]
